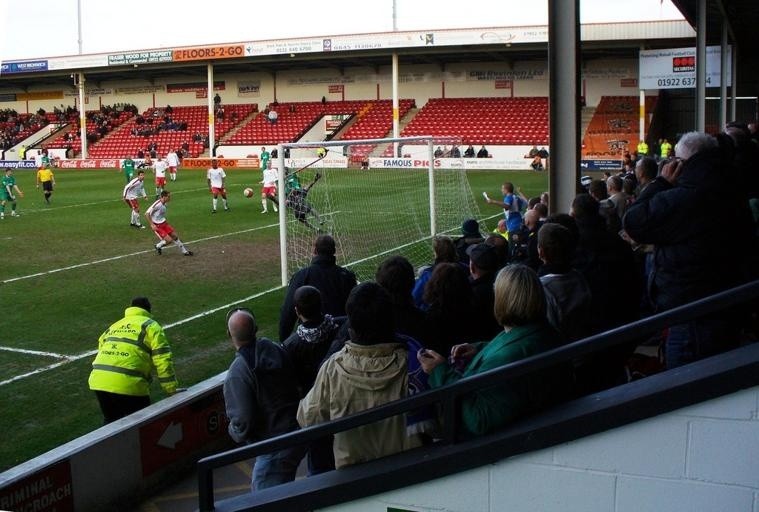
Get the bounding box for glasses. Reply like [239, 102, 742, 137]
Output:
[225, 305, 256, 327]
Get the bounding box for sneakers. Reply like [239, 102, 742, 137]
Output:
[260, 209, 269, 214]
[11, 212, 21, 217]
[153, 243, 162, 255]
[0, 215, 5, 219]
[272, 206, 278, 213]
[211, 209, 217, 216]
[224, 207, 231, 212]
[130, 223, 146, 229]
[319, 220, 326, 226]
[184, 250, 194, 257]
[317, 227, 328, 235]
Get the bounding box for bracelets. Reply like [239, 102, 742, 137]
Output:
[37, 184, 39, 185]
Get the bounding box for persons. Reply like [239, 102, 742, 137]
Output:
[122, 171, 147, 228]
[152, 153, 170, 197]
[2, 167, 25, 220]
[164, 148, 180, 181]
[130, 96, 238, 169]
[207, 159, 230, 214]
[86, 296, 187, 427]
[145, 191, 194, 256]
[226, 122, 758, 494]
[263, 99, 295, 122]
[37, 163, 55, 205]
[122, 156, 135, 180]
[2, 96, 131, 170]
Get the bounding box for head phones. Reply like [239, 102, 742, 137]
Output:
[225, 307, 258, 338]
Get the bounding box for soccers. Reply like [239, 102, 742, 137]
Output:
[244, 188, 253, 197]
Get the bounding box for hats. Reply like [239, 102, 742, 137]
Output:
[461, 219, 480, 235]
[465, 243, 497, 269]
